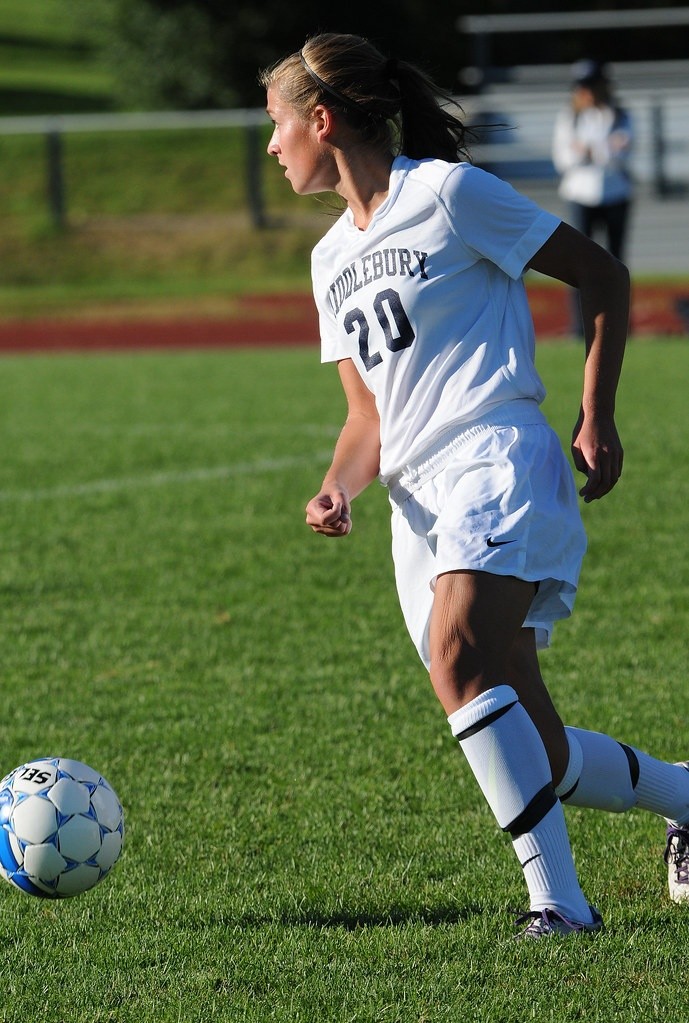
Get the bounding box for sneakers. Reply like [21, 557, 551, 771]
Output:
[664, 761, 689, 902]
[507, 905, 607, 943]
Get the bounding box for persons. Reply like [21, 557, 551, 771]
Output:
[264, 35, 689, 938]
[553, 59, 633, 343]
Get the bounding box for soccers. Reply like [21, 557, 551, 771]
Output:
[0, 757, 127, 902]
